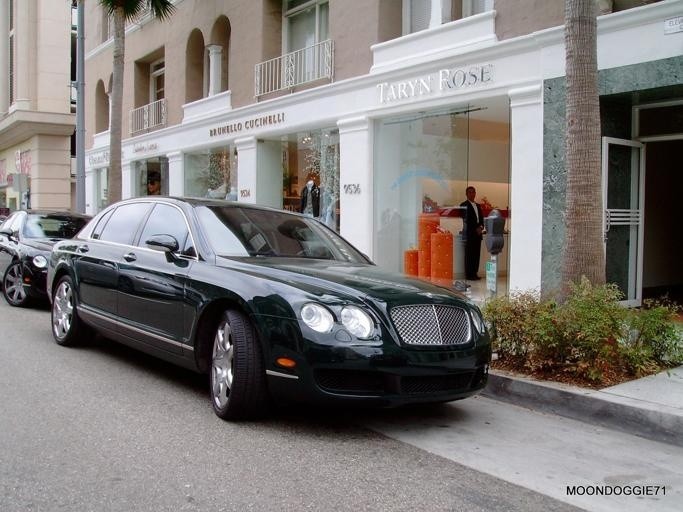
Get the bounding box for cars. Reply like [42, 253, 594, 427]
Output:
[0, 207, 99, 311]
[40, 189, 505, 430]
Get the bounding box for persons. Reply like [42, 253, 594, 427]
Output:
[147, 171, 161, 195]
[460, 186, 484, 281]
[300, 180, 320, 218]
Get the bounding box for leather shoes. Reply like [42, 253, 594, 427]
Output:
[466, 275, 481, 281]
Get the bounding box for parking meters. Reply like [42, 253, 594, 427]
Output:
[481, 208, 505, 301]
